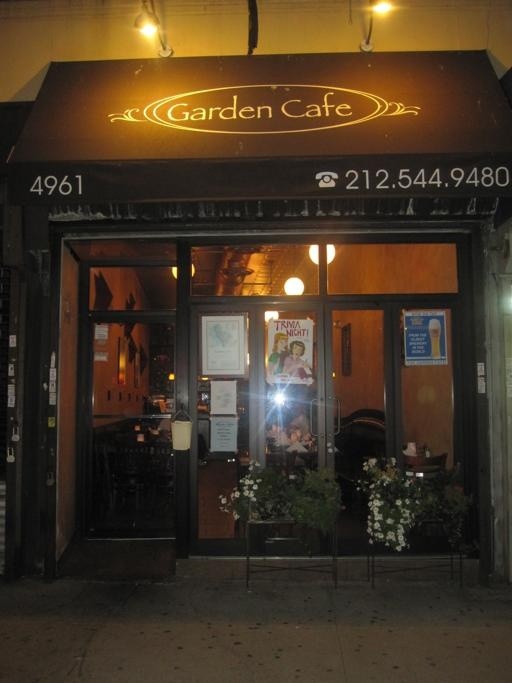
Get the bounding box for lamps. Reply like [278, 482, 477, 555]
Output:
[359, 0, 394, 54]
[132, 1, 173, 57]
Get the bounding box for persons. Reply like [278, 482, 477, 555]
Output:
[283, 340, 311, 381]
[268, 332, 289, 382]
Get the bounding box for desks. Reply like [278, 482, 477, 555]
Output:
[243, 517, 338, 590]
[368, 515, 463, 588]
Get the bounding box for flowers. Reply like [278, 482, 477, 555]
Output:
[217, 460, 341, 524]
[355, 457, 464, 553]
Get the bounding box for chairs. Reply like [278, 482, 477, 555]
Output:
[234, 451, 297, 539]
[394, 452, 448, 537]
[94, 418, 176, 539]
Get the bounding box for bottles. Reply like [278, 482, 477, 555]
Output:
[426, 448, 430, 458]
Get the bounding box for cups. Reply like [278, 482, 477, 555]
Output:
[407, 443, 417, 454]
